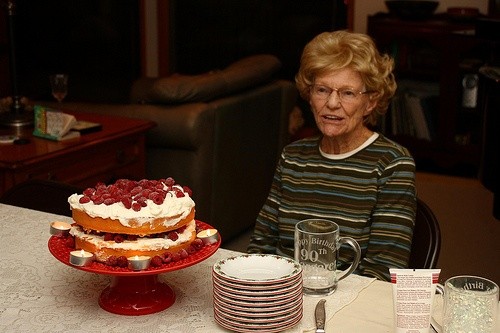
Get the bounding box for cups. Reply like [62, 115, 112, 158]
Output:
[430, 274, 500, 333]
[293, 219, 362, 298]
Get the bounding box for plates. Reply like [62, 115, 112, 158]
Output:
[212, 254, 303, 333]
[448, 7, 480, 16]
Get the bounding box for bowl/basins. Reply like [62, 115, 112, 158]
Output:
[384, 0, 439, 14]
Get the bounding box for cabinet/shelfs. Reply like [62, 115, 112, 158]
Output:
[367, 14, 473, 179]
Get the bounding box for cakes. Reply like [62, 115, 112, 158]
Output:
[68, 177, 203, 267]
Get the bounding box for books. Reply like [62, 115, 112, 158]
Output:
[373, 92, 440, 142]
[32, 105, 80, 141]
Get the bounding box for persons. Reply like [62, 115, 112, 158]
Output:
[247, 30, 415, 283]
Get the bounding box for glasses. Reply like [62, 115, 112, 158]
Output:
[309, 81, 375, 100]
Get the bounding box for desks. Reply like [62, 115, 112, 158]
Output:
[0, 202, 445, 333]
[0, 104, 156, 201]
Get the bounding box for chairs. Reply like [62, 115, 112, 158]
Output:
[410, 197, 442, 269]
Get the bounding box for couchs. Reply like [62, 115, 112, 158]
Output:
[30, 55, 297, 245]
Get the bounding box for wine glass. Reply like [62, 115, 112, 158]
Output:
[50, 74, 71, 111]
